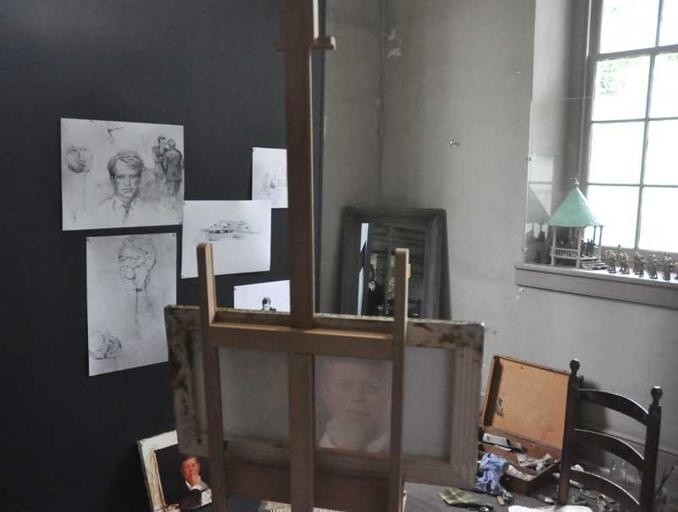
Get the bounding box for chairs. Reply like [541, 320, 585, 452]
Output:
[508, 359, 663, 512]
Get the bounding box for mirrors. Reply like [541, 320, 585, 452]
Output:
[340, 209, 446, 320]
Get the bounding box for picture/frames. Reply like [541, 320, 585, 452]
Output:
[164, 305, 485, 491]
[136, 428, 212, 512]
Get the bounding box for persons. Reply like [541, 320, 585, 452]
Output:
[368, 262, 384, 314]
[97, 135, 181, 222]
[319, 356, 392, 454]
[260, 297, 278, 314]
[168, 454, 207, 510]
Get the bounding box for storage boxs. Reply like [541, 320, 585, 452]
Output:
[478, 355, 584, 496]
